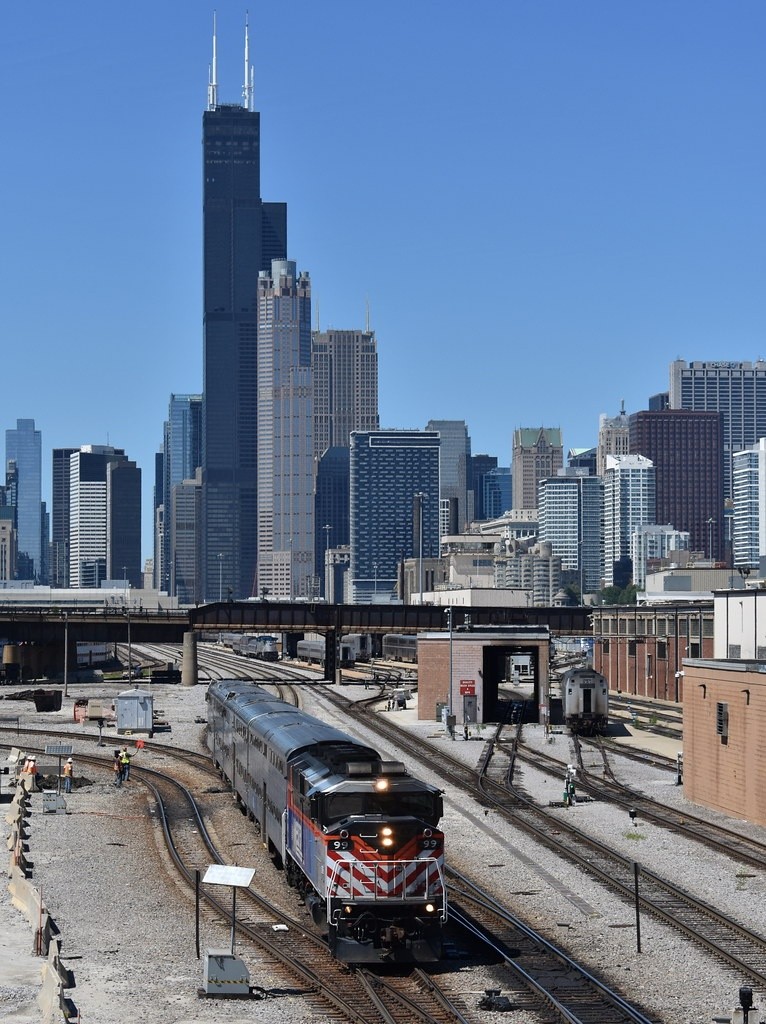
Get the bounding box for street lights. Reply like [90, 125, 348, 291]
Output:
[1, 542, 6, 580]
[579, 541, 585, 606]
[323, 525, 333, 604]
[706, 518, 717, 561]
[374, 562, 378, 594]
[415, 492, 427, 605]
[122, 567, 128, 603]
[59, 610, 68, 697]
[169, 561, 173, 596]
[288, 538, 294, 604]
[123, 612, 131, 685]
[444, 608, 453, 737]
[217, 553, 224, 602]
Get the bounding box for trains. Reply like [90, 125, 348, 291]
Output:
[205, 680, 449, 969]
[232, 636, 278, 662]
[383, 634, 418, 664]
[341, 634, 372, 663]
[297, 641, 356, 669]
[561, 668, 608, 735]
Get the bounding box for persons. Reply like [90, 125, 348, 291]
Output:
[113, 754, 123, 788]
[22, 755, 36, 791]
[62, 757, 74, 793]
[120, 748, 138, 781]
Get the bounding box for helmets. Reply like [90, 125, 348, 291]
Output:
[67, 757, 73, 762]
[27, 755, 36, 760]
[119, 753, 123, 757]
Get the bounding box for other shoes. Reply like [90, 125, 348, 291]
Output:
[113, 782, 122, 788]
[122, 778, 130, 781]
[66, 791, 72, 793]
